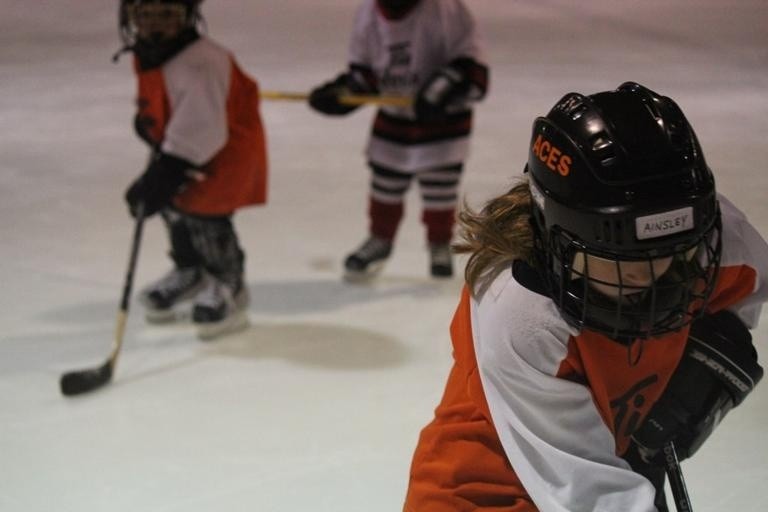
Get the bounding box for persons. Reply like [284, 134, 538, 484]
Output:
[401, 82, 767, 512]
[116, 1, 274, 323]
[306, 0, 489, 280]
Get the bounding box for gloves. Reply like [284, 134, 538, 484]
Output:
[310, 63, 381, 115]
[417, 55, 473, 125]
[624, 310, 763, 479]
[127, 144, 212, 217]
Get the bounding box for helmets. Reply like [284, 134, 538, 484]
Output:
[523, 82, 724, 342]
[118, 2, 199, 56]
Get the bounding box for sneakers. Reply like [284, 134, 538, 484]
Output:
[345, 237, 391, 270]
[194, 269, 242, 322]
[144, 263, 207, 312]
[432, 242, 451, 275]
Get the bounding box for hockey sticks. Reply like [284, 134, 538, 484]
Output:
[61, 145, 156, 394]
